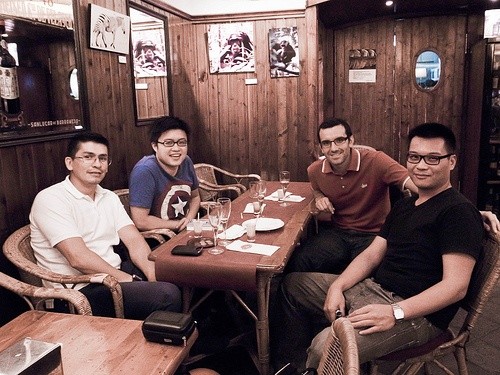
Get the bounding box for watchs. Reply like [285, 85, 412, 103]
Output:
[391, 303, 405, 325]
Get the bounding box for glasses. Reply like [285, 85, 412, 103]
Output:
[71, 154, 113, 166]
[156, 139, 187, 147]
[406, 153, 454, 165]
[320, 134, 352, 148]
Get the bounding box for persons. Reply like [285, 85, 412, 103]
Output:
[29, 131, 180, 321]
[269, 121, 486, 375]
[288, 118, 500, 274]
[129, 117, 208, 240]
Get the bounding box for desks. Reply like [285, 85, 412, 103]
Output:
[147, 181, 315, 375]
[0, 310, 199, 375]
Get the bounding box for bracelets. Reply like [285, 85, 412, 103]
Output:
[132, 274, 143, 281]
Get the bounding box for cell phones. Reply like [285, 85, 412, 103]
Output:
[171, 245, 203, 255]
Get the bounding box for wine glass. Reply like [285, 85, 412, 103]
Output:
[217, 199, 231, 246]
[279, 171, 291, 207]
[208, 202, 223, 255]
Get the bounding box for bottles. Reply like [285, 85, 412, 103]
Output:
[0, 34, 21, 116]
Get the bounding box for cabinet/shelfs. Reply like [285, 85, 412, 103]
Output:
[461, 39, 500, 221]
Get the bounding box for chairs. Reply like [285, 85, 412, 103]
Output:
[193, 163, 262, 203]
[317, 315, 360, 375]
[369, 210, 500, 375]
[0, 271, 93, 316]
[112, 188, 177, 239]
[1, 223, 166, 319]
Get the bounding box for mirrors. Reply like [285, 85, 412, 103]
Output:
[65, 64, 80, 104]
[0, 0, 91, 150]
[411, 48, 445, 94]
[126, 0, 175, 128]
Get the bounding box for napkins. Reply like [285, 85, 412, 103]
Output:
[243, 203, 266, 215]
[283, 194, 306, 203]
[263, 189, 292, 201]
[217, 224, 246, 240]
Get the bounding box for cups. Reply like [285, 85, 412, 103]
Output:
[246, 222, 256, 241]
[193, 223, 202, 238]
[253, 201, 260, 218]
[256, 186, 266, 206]
[249, 181, 260, 199]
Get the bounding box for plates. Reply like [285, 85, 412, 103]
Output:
[242, 216, 285, 232]
